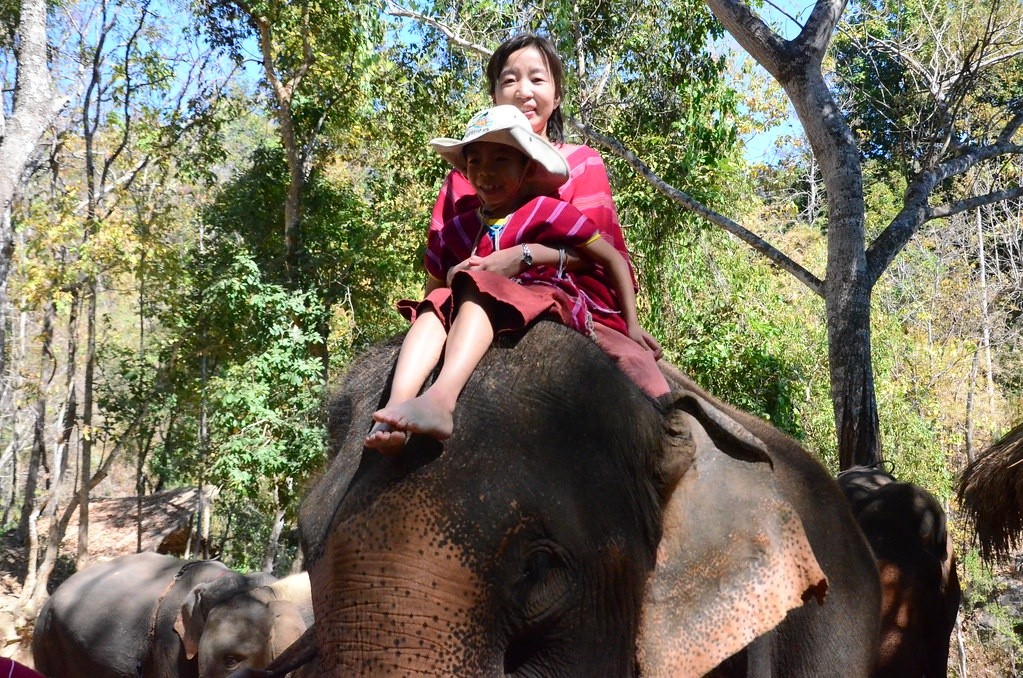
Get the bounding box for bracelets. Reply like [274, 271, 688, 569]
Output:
[557, 248, 568, 278]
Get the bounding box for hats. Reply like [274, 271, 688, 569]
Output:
[430, 104, 569, 196]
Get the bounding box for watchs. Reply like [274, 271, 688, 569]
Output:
[522, 243, 533, 268]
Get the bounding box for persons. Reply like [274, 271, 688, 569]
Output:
[425, 33, 675, 414]
[364, 104, 664, 460]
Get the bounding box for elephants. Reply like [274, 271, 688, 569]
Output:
[31, 302, 962, 678]
